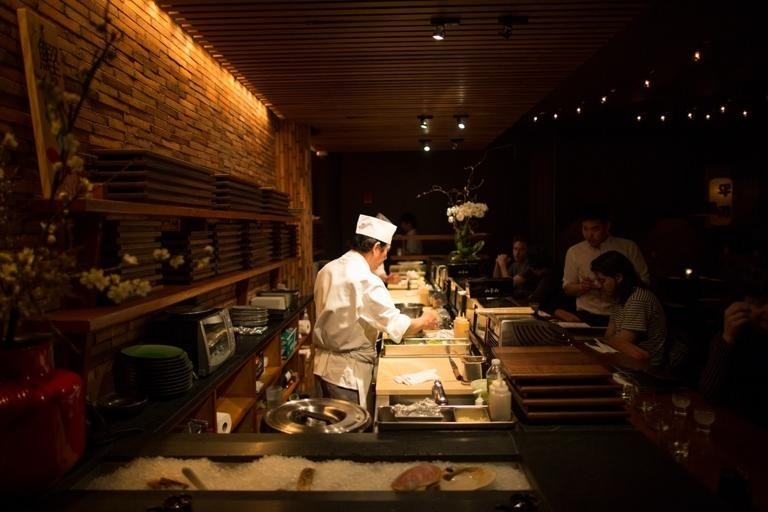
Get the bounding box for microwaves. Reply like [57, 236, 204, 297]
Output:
[143, 309, 236, 378]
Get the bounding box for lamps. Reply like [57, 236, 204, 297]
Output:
[423, 142, 432, 151]
[457, 118, 466, 130]
[432, 24, 445, 40]
[449, 143, 458, 151]
[497, 25, 513, 39]
[419, 119, 429, 129]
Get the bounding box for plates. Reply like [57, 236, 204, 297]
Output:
[229, 303, 270, 330]
[96, 342, 199, 416]
[425, 461, 500, 491]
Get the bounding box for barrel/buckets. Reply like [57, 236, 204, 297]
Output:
[395, 303, 424, 337]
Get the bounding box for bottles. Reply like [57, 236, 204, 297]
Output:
[453, 308, 470, 354]
[486, 357, 513, 423]
[431, 378, 449, 406]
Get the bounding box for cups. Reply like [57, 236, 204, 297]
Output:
[618, 376, 717, 464]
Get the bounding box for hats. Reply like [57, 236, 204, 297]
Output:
[355, 213, 398, 245]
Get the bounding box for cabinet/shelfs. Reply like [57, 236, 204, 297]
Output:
[87, 293, 316, 439]
[15, 9, 301, 332]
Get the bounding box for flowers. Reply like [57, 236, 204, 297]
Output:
[414, 149, 496, 263]
[0, 1, 214, 346]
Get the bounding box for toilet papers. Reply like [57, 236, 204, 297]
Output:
[298, 320, 311, 334]
[216, 412, 231, 434]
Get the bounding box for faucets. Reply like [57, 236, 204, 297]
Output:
[430, 380, 447, 404]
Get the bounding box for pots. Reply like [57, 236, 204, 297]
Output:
[259, 393, 374, 440]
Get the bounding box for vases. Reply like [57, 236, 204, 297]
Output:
[447, 220, 485, 265]
[0, 335, 87, 489]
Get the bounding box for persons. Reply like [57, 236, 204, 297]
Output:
[311, 214, 441, 415]
[509, 252, 557, 309]
[399, 211, 425, 255]
[491, 236, 531, 280]
[699, 272, 768, 433]
[372, 211, 402, 287]
[560, 209, 652, 327]
[554, 250, 670, 388]
